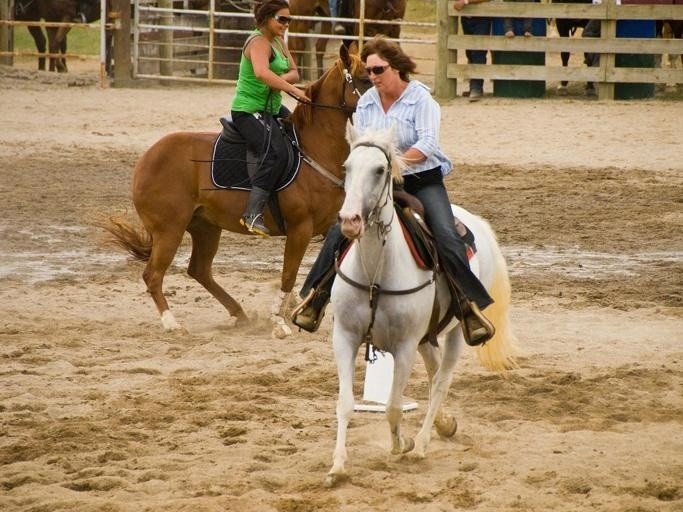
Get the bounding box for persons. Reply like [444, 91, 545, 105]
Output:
[289, 31, 491, 348]
[326, 1, 347, 34]
[502, 1, 538, 39]
[579, 0, 626, 89]
[450, 0, 491, 102]
[228, 1, 313, 240]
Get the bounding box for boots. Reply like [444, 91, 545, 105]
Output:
[238, 186, 271, 239]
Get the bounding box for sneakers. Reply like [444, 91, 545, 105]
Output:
[468, 87, 484, 103]
[294, 291, 327, 330]
[457, 301, 489, 342]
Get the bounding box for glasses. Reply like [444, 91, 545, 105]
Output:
[269, 14, 294, 25]
[362, 63, 393, 75]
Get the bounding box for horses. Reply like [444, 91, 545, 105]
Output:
[9, 0, 79, 74]
[172, 0, 333, 81]
[333, 0, 406, 53]
[321, 116, 524, 490]
[89, 40, 375, 340]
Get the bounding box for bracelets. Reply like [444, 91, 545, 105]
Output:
[463, 0, 469, 6]
